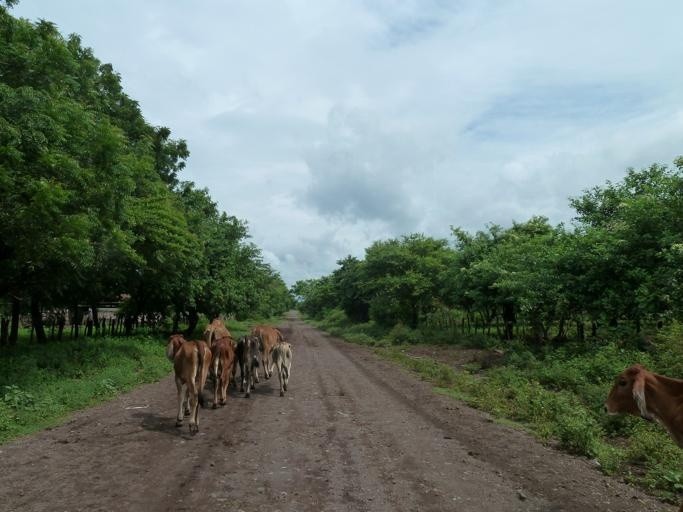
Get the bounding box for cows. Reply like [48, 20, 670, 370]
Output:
[165, 317, 293, 437]
[598, 362, 683, 452]
